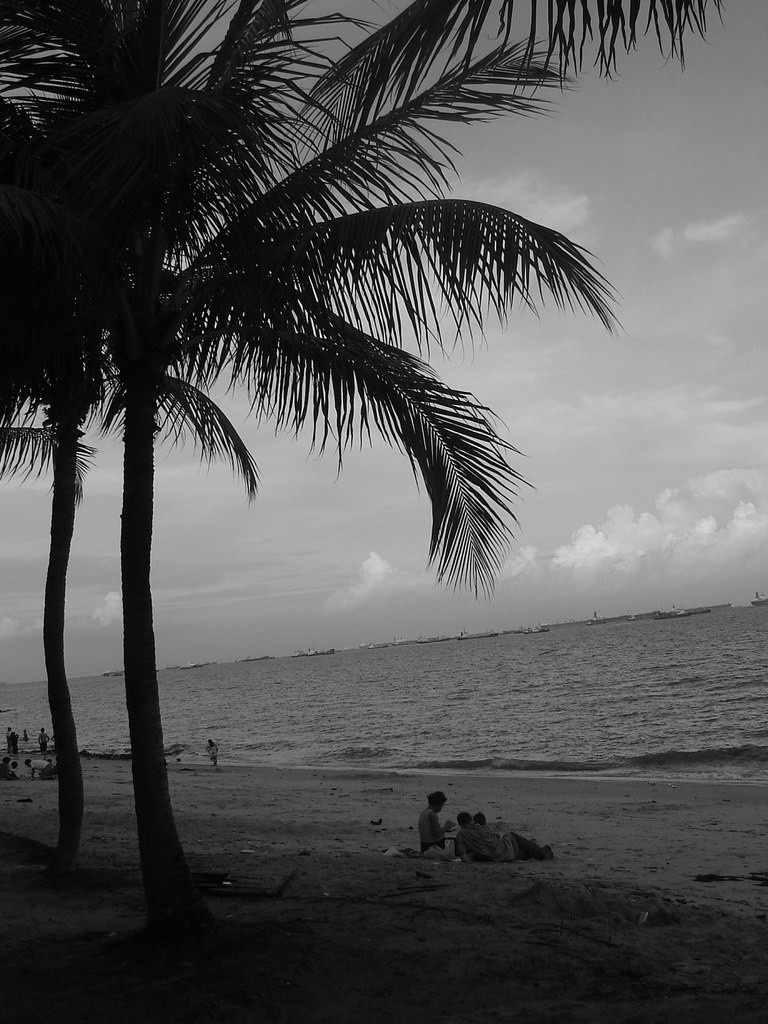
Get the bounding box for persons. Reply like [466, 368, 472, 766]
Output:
[417, 791, 456, 856]
[6, 728, 29, 755]
[38, 728, 50, 759]
[206, 739, 219, 764]
[0, 757, 59, 781]
[455, 810, 554, 864]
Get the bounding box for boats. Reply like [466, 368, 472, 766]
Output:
[585, 610, 607, 625]
[100, 647, 275, 679]
[751, 592, 768, 607]
[291, 620, 549, 661]
